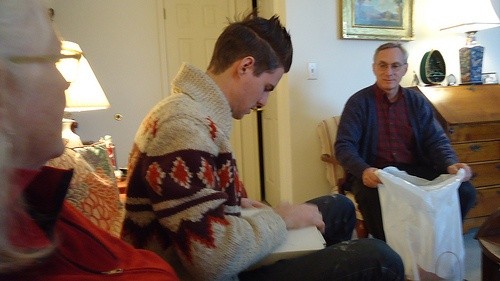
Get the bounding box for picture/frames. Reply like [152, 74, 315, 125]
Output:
[481, 73, 498, 84]
[341, 0, 414, 42]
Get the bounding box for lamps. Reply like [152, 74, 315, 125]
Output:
[440, 0, 500, 84]
[55, 40, 111, 148]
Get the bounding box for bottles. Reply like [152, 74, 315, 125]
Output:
[105, 135, 117, 170]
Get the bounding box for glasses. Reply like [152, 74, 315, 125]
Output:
[11, 45, 81, 67]
[377, 62, 403, 71]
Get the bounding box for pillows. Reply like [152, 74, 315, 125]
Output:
[43, 140, 124, 238]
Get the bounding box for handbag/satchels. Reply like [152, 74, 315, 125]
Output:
[420, 252, 467, 281]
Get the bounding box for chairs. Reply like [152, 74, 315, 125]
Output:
[318, 116, 369, 239]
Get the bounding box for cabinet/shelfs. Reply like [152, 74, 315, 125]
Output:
[421, 85, 500, 233]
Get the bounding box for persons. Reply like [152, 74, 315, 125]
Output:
[120, 12, 405, 281]
[330, 43, 478, 241]
[0, 0, 180, 281]
